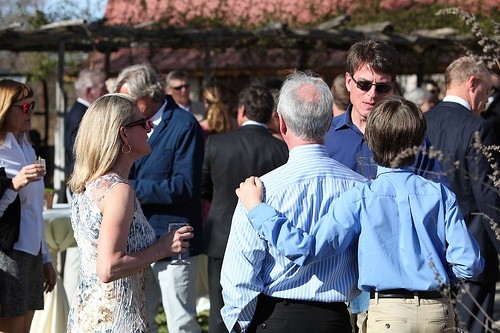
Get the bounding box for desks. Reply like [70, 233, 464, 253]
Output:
[30, 204, 78, 333]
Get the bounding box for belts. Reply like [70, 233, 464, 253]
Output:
[370, 289, 443, 299]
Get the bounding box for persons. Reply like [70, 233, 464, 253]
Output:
[64, 38, 500, 333]
[0, 79, 57, 333]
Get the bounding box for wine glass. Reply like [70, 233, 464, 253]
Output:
[168, 222, 191, 265]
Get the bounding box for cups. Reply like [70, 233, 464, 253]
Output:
[34, 158, 46, 174]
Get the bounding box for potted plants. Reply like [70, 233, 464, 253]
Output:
[44, 188, 56, 209]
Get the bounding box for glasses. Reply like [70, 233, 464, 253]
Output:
[468, 76, 495, 96]
[10, 101, 36, 113]
[352, 74, 394, 93]
[171, 84, 189, 90]
[125, 118, 151, 133]
